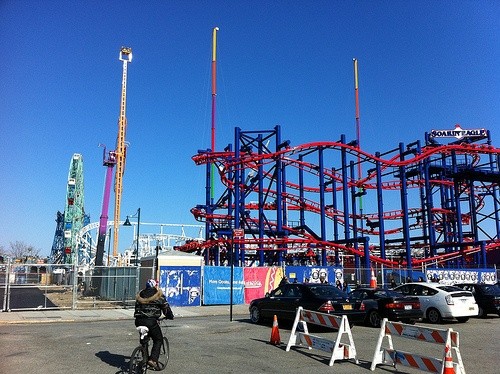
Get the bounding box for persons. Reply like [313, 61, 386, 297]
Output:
[343, 281, 351, 297]
[279, 277, 309, 286]
[336, 280, 342, 290]
[419, 277, 423, 282]
[391, 279, 397, 288]
[430, 275, 440, 283]
[320, 276, 327, 284]
[134, 279, 174, 367]
[377, 273, 382, 284]
[407, 276, 412, 283]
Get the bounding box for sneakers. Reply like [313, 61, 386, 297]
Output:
[148, 359, 158, 369]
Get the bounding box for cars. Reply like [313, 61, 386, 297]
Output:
[453, 284, 500, 318]
[388, 282, 480, 324]
[249, 283, 367, 332]
[346, 287, 424, 328]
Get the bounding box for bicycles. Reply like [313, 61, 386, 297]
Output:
[129, 316, 170, 374]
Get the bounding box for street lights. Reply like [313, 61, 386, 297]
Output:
[123, 208, 140, 266]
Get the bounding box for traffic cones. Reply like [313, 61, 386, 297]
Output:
[369, 267, 377, 288]
[269, 315, 281, 345]
[443, 345, 457, 374]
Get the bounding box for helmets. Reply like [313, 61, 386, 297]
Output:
[146, 280, 156, 287]
